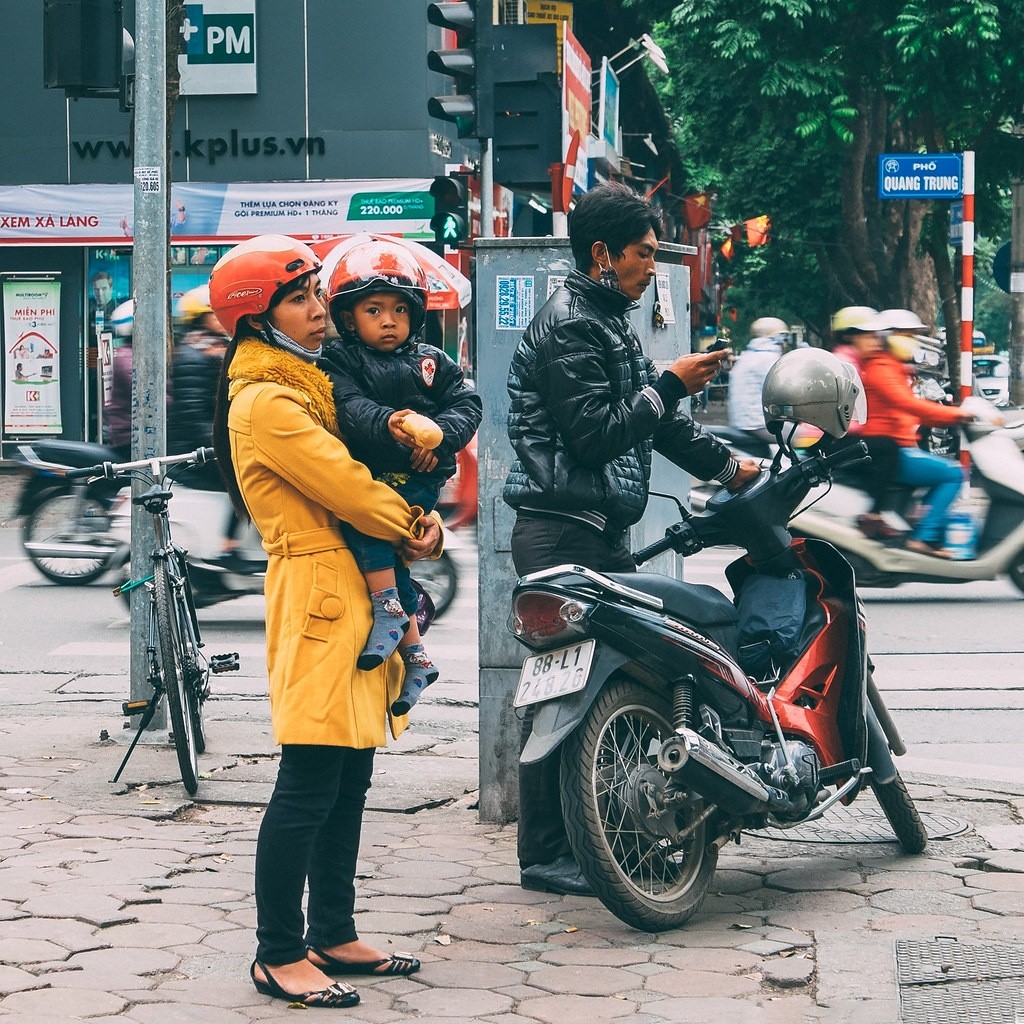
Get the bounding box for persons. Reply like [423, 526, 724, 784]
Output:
[167, 290, 266, 576]
[105, 300, 172, 461]
[90, 272, 119, 323]
[729, 317, 797, 445]
[795, 307, 975, 559]
[210, 233, 447, 1006]
[502, 182, 762, 893]
[317, 241, 483, 716]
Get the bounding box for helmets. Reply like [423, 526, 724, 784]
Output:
[751, 317, 792, 339]
[178, 285, 214, 321]
[884, 309, 928, 329]
[209, 234, 323, 338]
[762, 347, 868, 438]
[327, 239, 430, 341]
[109, 300, 134, 336]
[832, 306, 888, 331]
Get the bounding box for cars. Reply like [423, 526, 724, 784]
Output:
[972, 329, 1010, 409]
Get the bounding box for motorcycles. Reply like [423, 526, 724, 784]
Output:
[504, 422, 930, 935]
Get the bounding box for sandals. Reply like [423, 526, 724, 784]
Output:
[250, 959, 360, 1007]
[306, 942, 420, 975]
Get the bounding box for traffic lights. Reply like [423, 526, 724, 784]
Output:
[428, 175, 469, 244]
[426, 0, 496, 140]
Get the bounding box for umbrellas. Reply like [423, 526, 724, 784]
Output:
[311, 228, 472, 309]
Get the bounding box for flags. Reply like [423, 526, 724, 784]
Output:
[747, 216, 771, 245]
[686, 193, 712, 229]
[721, 226, 741, 260]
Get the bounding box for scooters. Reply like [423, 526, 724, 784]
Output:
[688, 395, 1024, 598]
[11, 436, 463, 620]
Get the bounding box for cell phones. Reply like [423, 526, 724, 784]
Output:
[708, 338, 728, 354]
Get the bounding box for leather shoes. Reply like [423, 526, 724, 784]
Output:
[626, 847, 683, 879]
[521, 855, 596, 897]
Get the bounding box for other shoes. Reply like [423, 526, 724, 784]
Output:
[906, 540, 954, 558]
[858, 514, 903, 540]
[218, 547, 247, 559]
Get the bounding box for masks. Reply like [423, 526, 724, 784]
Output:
[597, 243, 621, 292]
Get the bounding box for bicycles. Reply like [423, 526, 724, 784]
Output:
[66, 447, 240, 798]
